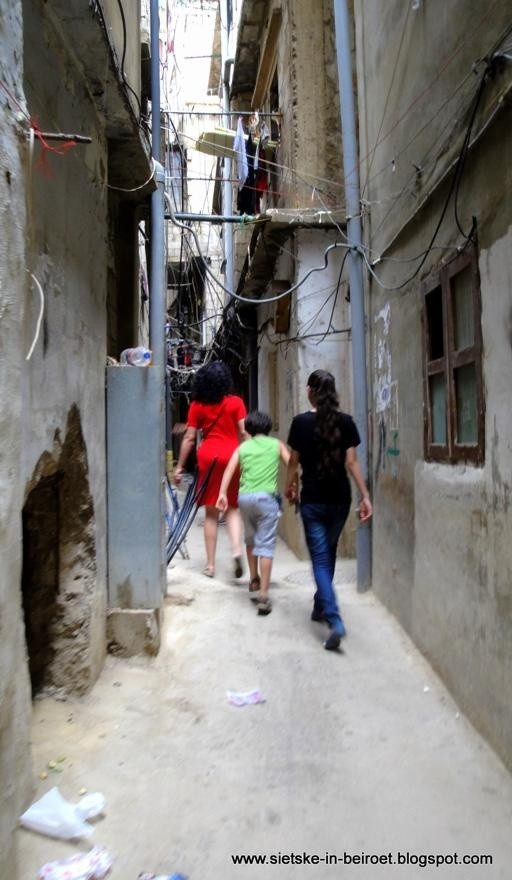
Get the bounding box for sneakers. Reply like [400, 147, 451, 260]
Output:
[311, 591, 324, 621]
[257, 598, 273, 616]
[322, 626, 344, 649]
[201, 564, 217, 578]
[231, 553, 244, 579]
[247, 574, 261, 601]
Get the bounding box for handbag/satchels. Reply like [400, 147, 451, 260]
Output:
[184, 447, 198, 474]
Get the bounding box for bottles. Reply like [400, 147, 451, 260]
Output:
[120, 347, 152, 368]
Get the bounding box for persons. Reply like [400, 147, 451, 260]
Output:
[215, 407, 298, 616]
[172, 360, 254, 579]
[284, 370, 374, 651]
